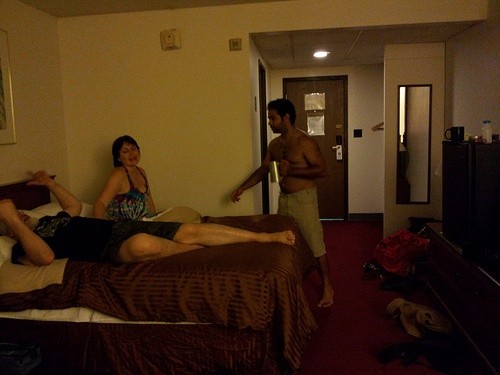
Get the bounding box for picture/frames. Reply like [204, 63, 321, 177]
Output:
[0, 29, 16, 145]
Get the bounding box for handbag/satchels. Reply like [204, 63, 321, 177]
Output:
[0, 342, 42, 375]
[374, 228, 430, 276]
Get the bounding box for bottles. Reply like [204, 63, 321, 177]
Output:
[482, 120, 492, 143]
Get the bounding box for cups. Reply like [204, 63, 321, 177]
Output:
[268, 160, 284, 183]
[444, 126, 464, 142]
[469, 135, 482, 142]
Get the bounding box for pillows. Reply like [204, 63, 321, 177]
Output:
[0, 202, 94, 259]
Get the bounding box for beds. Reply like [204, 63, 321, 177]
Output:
[0, 214, 319, 375]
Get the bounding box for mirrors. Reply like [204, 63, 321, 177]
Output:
[396, 84, 433, 205]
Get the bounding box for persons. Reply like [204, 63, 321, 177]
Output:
[92, 135, 156, 221]
[231, 99, 334, 307]
[0, 171, 295, 266]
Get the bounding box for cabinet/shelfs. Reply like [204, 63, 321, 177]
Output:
[442, 140, 500, 249]
